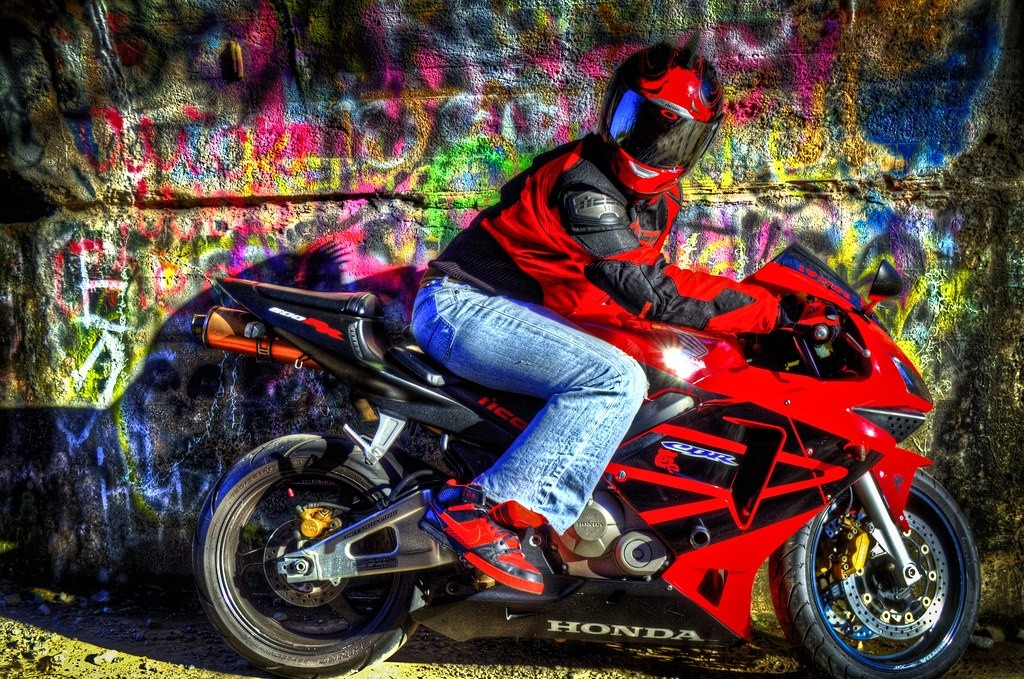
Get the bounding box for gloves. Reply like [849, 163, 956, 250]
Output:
[781, 293, 840, 343]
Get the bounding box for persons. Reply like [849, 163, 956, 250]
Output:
[409, 50, 844, 594]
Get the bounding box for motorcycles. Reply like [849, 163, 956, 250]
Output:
[185, 259, 981, 679]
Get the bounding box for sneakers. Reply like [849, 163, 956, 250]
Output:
[418, 477, 547, 596]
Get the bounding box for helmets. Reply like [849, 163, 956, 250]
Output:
[597, 42, 724, 198]
